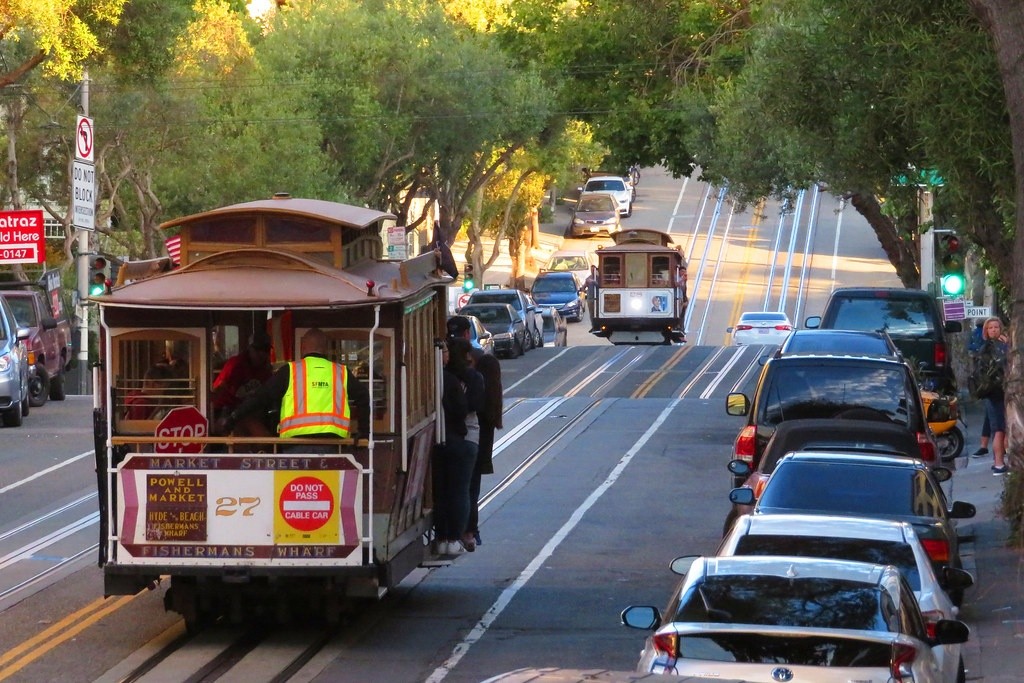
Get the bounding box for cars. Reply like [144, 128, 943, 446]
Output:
[448, 289, 545, 359]
[545, 250, 596, 300]
[619, 554, 973, 683]
[0, 293, 32, 429]
[756, 328, 908, 362]
[729, 448, 978, 610]
[523, 270, 586, 323]
[720, 419, 954, 538]
[569, 192, 623, 239]
[668, 514, 975, 683]
[725, 354, 946, 490]
[726, 312, 794, 347]
[576, 165, 641, 218]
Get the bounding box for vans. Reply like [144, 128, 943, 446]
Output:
[803, 286, 963, 397]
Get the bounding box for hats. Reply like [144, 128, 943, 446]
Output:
[445, 315, 471, 337]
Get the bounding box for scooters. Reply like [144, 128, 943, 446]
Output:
[912, 381, 965, 463]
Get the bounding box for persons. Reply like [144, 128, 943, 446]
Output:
[124, 362, 173, 454]
[980, 316, 1009, 476]
[679, 266, 686, 275]
[651, 296, 662, 312]
[446, 316, 503, 545]
[967, 318, 986, 400]
[971, 416, 1008, 458]
[211, 330, 270, 422]
[580, 265, 599, 333]
[443, 341, 484, 552]
[434, 338, 467, 555]
[255, 329, 365, 454]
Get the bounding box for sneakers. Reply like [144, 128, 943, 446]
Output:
[447, 541, 467, 554]
[437, 541, 447, 554]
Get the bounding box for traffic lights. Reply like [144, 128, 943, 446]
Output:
[463, 263, 474, 294]
[940, 235, 968, 300]
[89, 254, 108, 297]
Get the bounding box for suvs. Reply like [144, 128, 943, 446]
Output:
[0, 269, 75, 408]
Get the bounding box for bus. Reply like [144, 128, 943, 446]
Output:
[86, 192, 461, 638]
[589, 229, 690, 347]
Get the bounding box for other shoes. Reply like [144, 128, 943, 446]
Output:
[972, 447, 989, 458]
[473, 531, 482, 545]
[993, 467, 1011, 476]
[991, 462, 1005, 469]
[462, 538, 477, 552]
